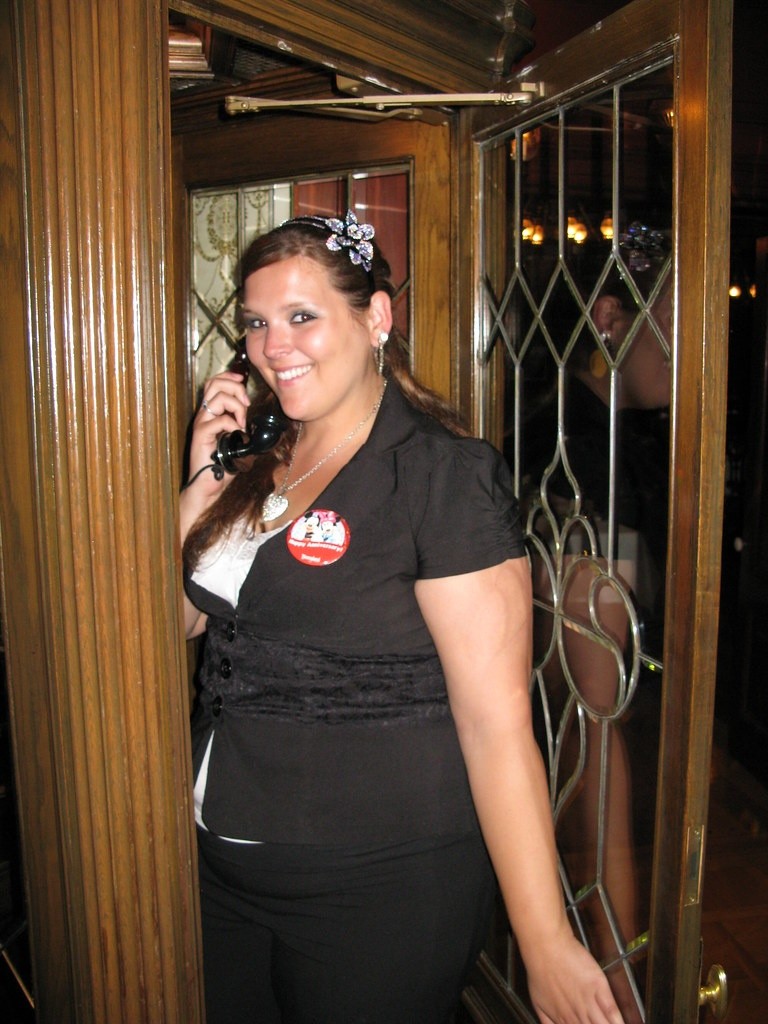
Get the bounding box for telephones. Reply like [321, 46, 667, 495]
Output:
[215, 335, 288, 476]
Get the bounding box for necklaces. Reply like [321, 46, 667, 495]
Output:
[263, 384, 387, 522]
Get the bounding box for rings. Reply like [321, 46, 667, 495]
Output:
[201, 401, 213, 415]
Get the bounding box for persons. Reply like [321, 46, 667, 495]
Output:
[179, 210, 624, 1024]
[507, 221, 672, 1024]
